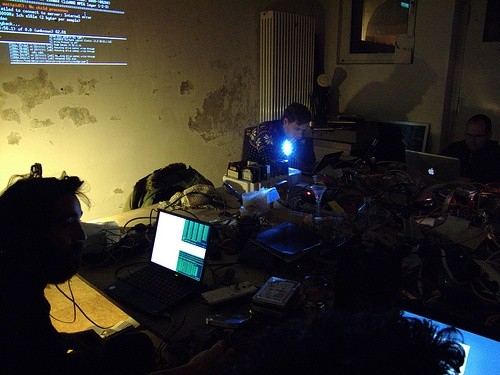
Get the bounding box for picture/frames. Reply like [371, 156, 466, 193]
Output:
[336, 0, 417, 64]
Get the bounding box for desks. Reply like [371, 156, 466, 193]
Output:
[73, 174, 407, 344]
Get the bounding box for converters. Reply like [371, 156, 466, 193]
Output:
[200, 280, 258, 311]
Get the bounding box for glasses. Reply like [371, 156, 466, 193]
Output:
[465, 132, 486, 137]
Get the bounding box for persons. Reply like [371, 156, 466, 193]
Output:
[244, 102, 317, 178]
[435, 113, 500, 186]
[0, 176, 235, 375]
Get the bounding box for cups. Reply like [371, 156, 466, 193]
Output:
[305, 275, 328, 309]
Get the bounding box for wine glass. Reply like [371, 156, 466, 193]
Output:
[310, 186, 327, 220]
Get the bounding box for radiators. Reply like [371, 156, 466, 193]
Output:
[260, 11, 315, 126]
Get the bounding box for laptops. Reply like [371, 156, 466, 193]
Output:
[104, 209, 214, 318]
[303, 151, 343, 176]
[405, 150, 460, 188]
[397, 309, 500, 375]
